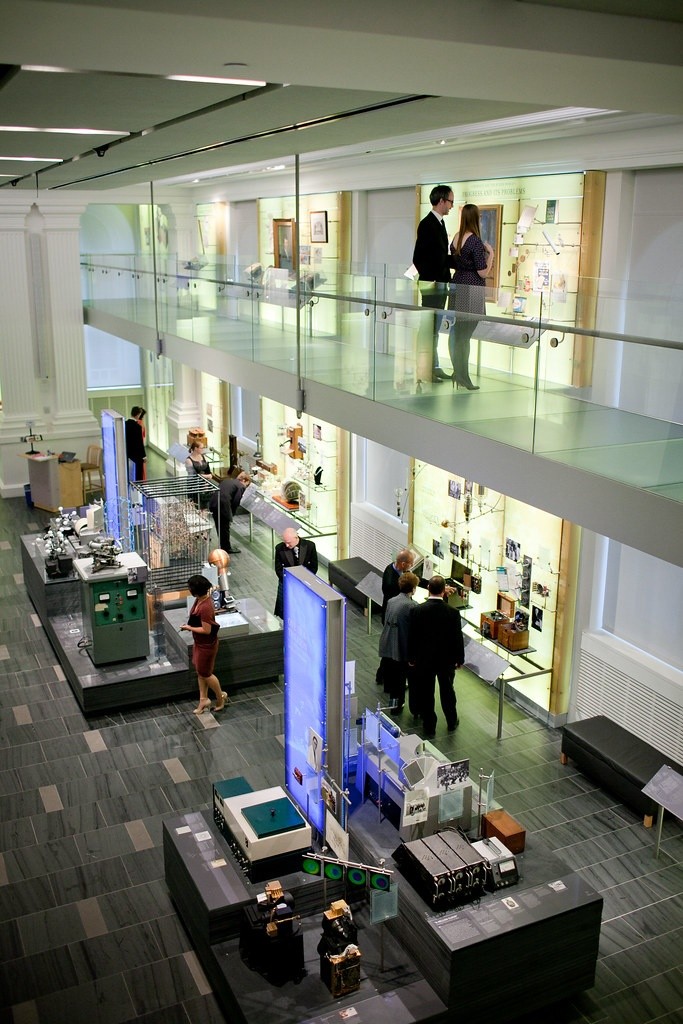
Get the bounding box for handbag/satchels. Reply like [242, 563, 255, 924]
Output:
[188, 613, 220, 639]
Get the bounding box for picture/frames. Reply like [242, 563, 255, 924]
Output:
[272, 218, 296, 271]
[309, 211, 328, 243]
[459, 204, 502, 303]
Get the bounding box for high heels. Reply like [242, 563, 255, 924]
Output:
[214, 691, 229, 711]
[192, 698, 212, 715]
[451, 370, 480, 390]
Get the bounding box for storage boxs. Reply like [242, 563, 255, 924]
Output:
[482, 592, 529, 651]
[482, 809, 526, 854]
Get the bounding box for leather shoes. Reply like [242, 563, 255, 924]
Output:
[416, 368, 453, 383]
[228, 546, 241, 554]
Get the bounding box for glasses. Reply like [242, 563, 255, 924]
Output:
[443, 195, 455, 204]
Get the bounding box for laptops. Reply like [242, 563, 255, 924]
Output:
[59, 452, 79, 463]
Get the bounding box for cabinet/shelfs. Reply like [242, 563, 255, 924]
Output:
[211, 467, 251, 514]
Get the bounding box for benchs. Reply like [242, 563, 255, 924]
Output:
[562, 714, 683, 828]
[328, 556, 384, 617]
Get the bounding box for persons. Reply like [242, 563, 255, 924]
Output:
[446, 204, 494, 391]
[309, 736, 318, 771]
[274, 528, 318, 619]
[411, 576, 465, 736]
[509, 541, 516, 561]
[439, 765, 467, 786]
[185, 441, 212, 509]
[412, 185, 455, 383]
[450, 481, 459, 499]
[125, 406, 148, 483]
[375, 550, 457, 715]
[209, 472, 252, 554]
[180, 575, 228, 714]
[533, 608, 539, 628]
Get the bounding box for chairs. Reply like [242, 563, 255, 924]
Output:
[80, 445, 106, 505]
